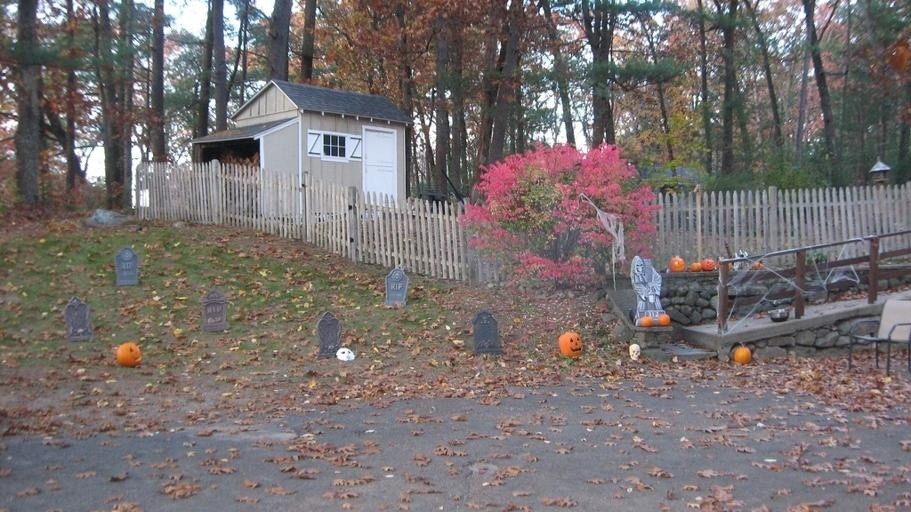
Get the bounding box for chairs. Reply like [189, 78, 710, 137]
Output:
[849, 299, 911, 376]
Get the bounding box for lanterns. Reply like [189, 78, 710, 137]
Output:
[702, 258, 716, 271]
[558, 332, 583, 358]
[117, 342, 142, 367]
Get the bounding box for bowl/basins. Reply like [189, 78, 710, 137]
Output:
[768, 307, 791, 321]
